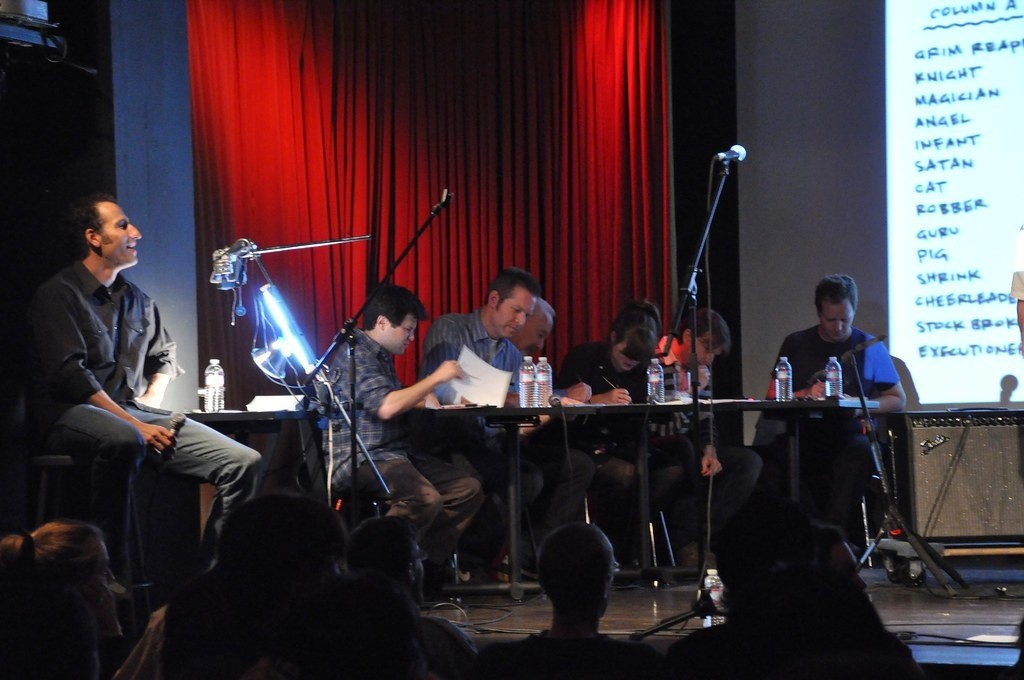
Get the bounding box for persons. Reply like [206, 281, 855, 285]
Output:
[321, 287, 484, 607]
[422, 269, 908, 581]
[0, 484, 927, 680]
[27, 192, 264, 636]
[1010, 270, 1024, 359]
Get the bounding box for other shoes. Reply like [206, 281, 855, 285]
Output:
[503, 552, 541, 580]
[677, 540, 717, 571]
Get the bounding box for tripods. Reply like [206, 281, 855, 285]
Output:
[628, 160, 729, 643]
[850, 354, 968, 598]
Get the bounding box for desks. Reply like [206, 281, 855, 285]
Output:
[185, 398, 880, 601]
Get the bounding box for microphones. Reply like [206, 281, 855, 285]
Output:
[548, 394, 562, 408]
[840, 335, 887, 364]
[158, 412, 186, 474]
[714, 145, 747, 162]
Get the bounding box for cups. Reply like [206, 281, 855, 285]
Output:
[673, 372, 690, 399]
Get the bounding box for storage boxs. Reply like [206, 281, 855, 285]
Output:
[882, 408, 1024, 543]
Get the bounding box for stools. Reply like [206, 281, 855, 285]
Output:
[30, 454, 149, 637]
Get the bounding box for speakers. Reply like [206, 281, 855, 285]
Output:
[882, 409, 1024, 550]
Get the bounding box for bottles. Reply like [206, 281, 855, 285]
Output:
[776, 357, 793, 401]
[203, 359, 225, 413]
[646, 358, 664, 402]
[519, 356, 553, 408]
[702, 569, 724, 628]
[825, 356, 843, 398]
